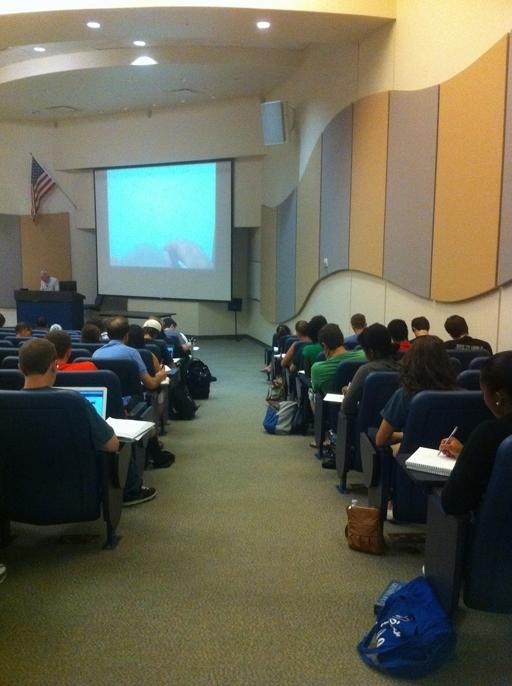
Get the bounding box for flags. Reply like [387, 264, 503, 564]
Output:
[29, 156, 61, 223]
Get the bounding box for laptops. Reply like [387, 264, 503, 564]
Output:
[167, 344, 175, 359]
[52, 386, 107, 421]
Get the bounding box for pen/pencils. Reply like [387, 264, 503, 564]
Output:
[347, 382, 352, 390]
[437, 426, 458, 456]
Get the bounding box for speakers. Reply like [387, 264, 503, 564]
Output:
[261, 101, 285, 145]
[228, 298, 242, 312]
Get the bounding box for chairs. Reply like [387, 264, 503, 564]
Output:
[265, 332, 512, 618]
[0, 325, 186, 552]
[84, 294, 103, 318]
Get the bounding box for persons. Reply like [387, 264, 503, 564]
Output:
[1, 312, 203, 510]
[261, 313, 495, 509]
[437, 349, 512, 581]
[38, 268, 60, 291]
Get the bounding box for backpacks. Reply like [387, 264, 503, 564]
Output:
[182, 359, 216, 399]
[173, 382, 199, 420]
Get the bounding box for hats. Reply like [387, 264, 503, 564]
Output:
[143, 319, 161, 332]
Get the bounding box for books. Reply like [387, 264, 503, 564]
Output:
[402, 443, 460, 478]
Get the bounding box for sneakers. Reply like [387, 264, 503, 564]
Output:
[123, 486, 155, 507]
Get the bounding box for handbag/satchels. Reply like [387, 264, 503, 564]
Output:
[358, 577, 453, 678]
[265, 385, 285, 401]
[263, 400, 297, 434]
[293, 408, 308, 434]
[345, 504, 384, 555]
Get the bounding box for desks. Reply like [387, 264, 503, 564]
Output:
[98, 309, 177, 320]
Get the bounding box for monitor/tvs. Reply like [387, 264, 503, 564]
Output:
[58, 280, 77, 292]
